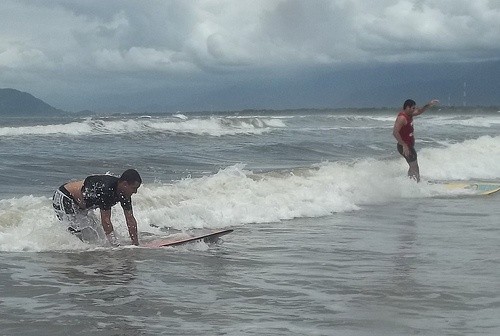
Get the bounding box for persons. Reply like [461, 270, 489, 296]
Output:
[53, 169, 143, 248]
[394, 98, 440, 184]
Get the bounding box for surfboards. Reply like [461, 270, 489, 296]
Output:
[427, 180, 500, 195]
[141, 229, 233, 247]
